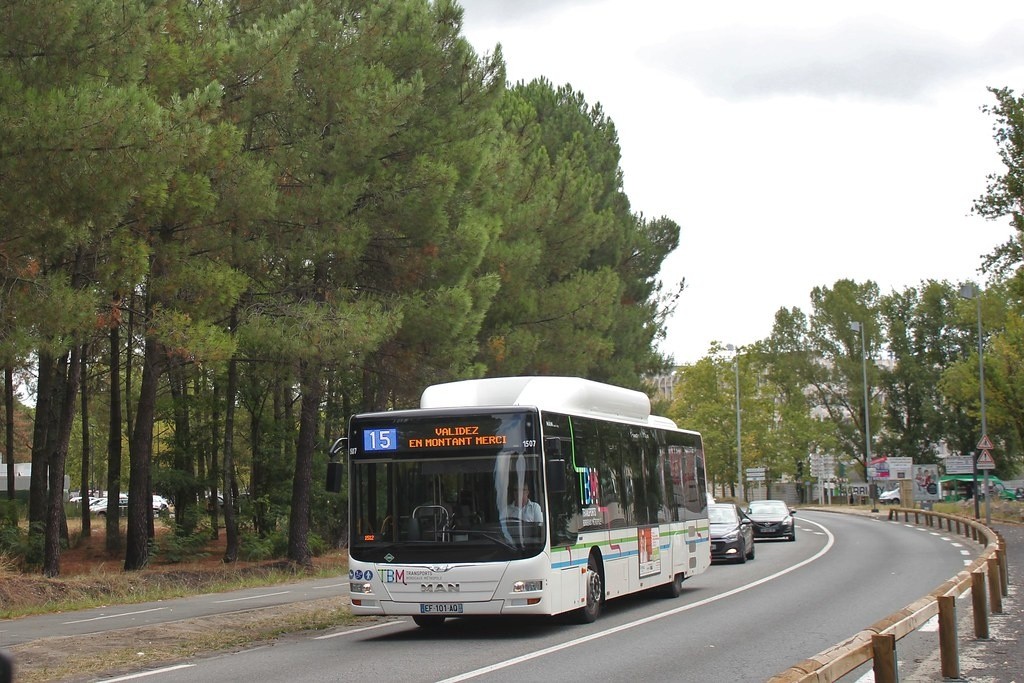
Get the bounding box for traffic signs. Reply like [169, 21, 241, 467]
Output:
[747, 476, 766, 481]
[745, 467, 765, 473]
[747, 472, 765, 477]
[808, 453, 835, 478]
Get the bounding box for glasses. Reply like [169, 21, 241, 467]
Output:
[514, 488, 526, 491]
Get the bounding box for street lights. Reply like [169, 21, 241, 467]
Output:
[959, 285, 992, 526]
[725, 343, 743, 505]
[848, 320, 873, 485]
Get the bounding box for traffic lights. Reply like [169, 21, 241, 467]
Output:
[796, 460, 803, 477]
[869, 484, 878, 498]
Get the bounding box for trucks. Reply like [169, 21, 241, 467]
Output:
[938, 473, 1016, 502]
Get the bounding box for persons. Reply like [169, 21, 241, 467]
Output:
[915, 468, 936, 491]
[499, 480, 544, 527]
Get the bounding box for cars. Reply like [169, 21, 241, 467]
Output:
[708, 504, 756, 564]
[744, 499, 798, 541]
[70, 488, 170, 521]
[880, 486, 901, 504]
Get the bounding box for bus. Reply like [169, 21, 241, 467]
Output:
[324, 376, 712, 628]
